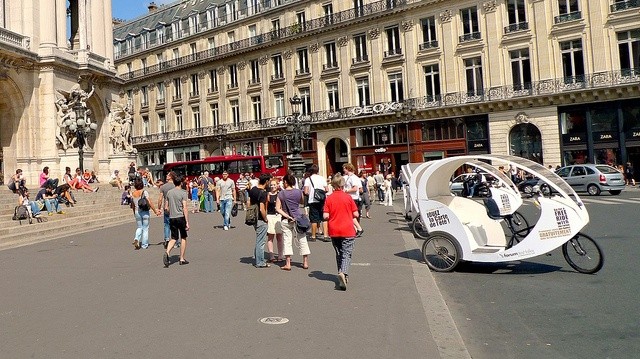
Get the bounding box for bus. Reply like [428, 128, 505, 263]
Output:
[164, 152, 286, 202]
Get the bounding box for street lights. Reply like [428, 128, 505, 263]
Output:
[64, 96, 97, 176]
[285, 95, 311, 183]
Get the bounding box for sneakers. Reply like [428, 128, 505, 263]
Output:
[192, 211, 194, 213]
[345, 276, 349, 283]
[48, 211, 54, 216]
[164, 239, 169, 248]
[38, 214, 43, 216]
[94, 187, 99, 192]
[173, 240, 180, 248]
[134, 238, 139, 249]
[366, 215, 372, 219]
[360, 213, 362, 217]
[223, 226, 228, 230]
[83, 189, 91, 193]
[228, 224, 231, 229]
[35, 215, 41, 218]
[196, 211, 199, 212]
[337, 272, 347, 291]
[57, 210, 65, 214]
[256, 263, 270, 267]
[264, 259, 269, 262]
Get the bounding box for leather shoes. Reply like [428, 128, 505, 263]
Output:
[356, 229, 364, 237]
[179, 259, 189, 265]
[163, 252, 169, 268]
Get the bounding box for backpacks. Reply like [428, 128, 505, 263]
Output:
[12, 205, 33, 225]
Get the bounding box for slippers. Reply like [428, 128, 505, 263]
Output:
[277, 259, 283, 262]
[300, 263, 308, 269]
[324, 236, 331, 241]
[306, 235, 316, 239]
[280, 265, 291, 269]
[269, 258, 278, 262]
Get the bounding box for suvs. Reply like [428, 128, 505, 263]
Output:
[556, 163, 625, 195]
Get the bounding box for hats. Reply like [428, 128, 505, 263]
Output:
[245, 172, 249, 176]
[191, 183, 198, 186]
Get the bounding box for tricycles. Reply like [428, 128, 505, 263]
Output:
[401, 153, 603, 273]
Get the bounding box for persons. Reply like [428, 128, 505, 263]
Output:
[121, 186, 131, 205]
[213, 171, 236, 231]
[235, 172, 260, 210]
[357, 170, 372, 219]
[159, 170, 181, 249]
[274, 173, 311, 270]
[130, 178, 160, 249]
[299, 164, 335, 242]
[7, 168, 26, 193]
[39, 167, 59, 188]
[127, 162, 157, 188]
[321, 173, 359, 290]
[108, 170, 123, 190]
[367, 170, 402, 206]
[62, 167, 99, 193]
[18, 187, 48, 223]
[607, 159, 636, 186]
[54, 183, 77, 207]
[342, 163, 364, 237]
[265, 177, 285, 263]
[463, 167, 487, 198]
[246, 173, 271, 268]
[35, 185, 65, 215]
[54, 82, 98, 151]
[107, 98, 137, 153]
[162, 177, 190, 267]
[187, 171, 214, 214]
[548, 165, 561, 171]
[498, 164, 526, 180]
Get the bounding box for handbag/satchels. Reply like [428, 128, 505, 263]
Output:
[138, 190, 150, 211]
[231, 203, 238, 217]
[281, 190, 311, 234]
[309, 175, 325, 201]
[245, 190, 264, 225]
[203, 175, 215, 192]
